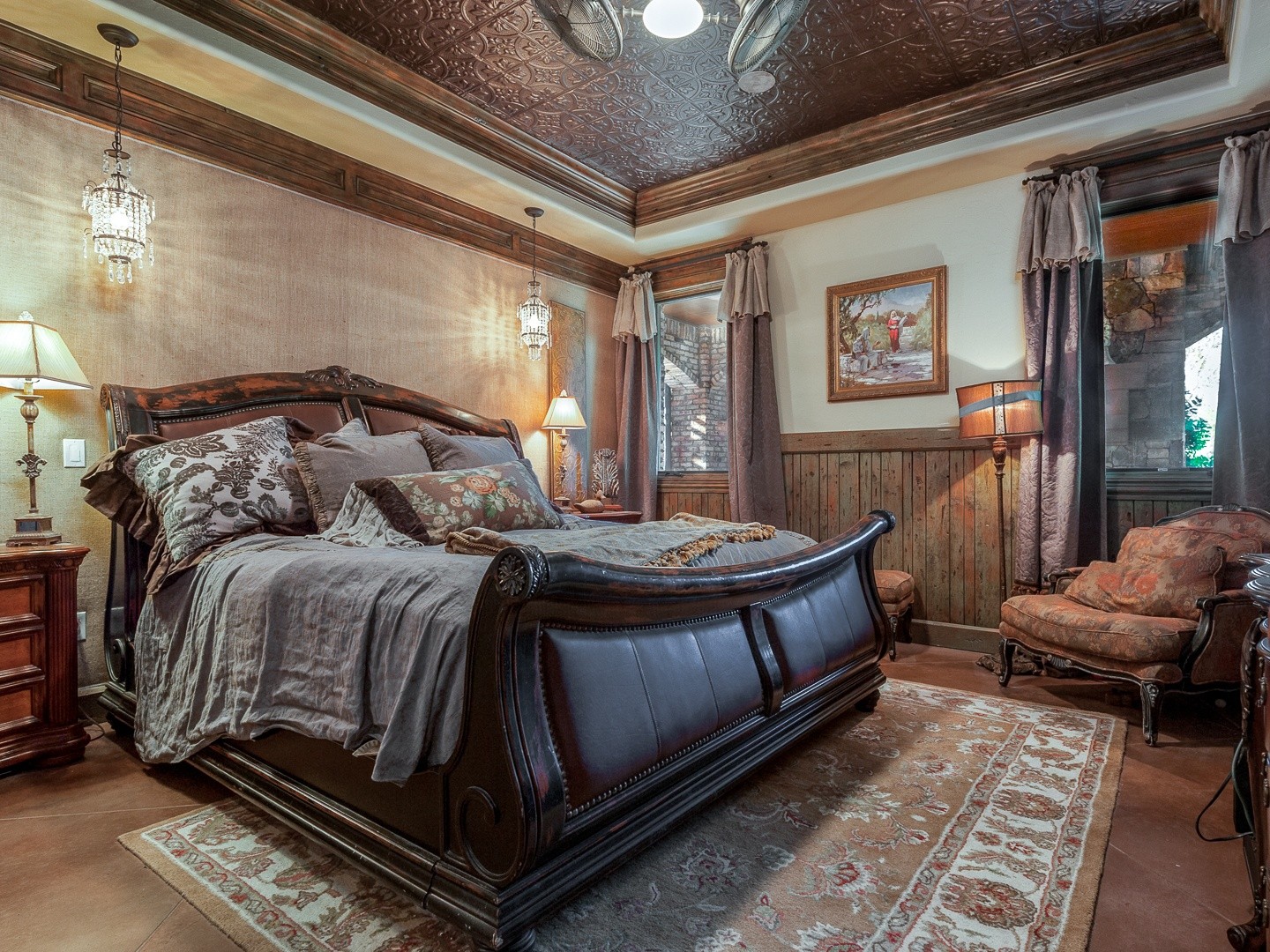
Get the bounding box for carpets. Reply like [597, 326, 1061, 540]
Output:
[115, 677, 1125, 952]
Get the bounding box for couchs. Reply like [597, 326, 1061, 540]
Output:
[997, 506, 1269, 747]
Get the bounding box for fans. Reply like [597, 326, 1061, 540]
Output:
[532, 0, 644, 65]
[727, 0, 810, 74]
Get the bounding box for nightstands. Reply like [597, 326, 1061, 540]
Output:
[0, 543, 92, 775]
[571, 510, 644, 524]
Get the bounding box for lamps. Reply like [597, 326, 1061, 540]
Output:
[80, 22, 157, 284]
[516, 207, 553, 362]
[955, 379, 1044, 675]
[541, 389, 588, 507]
[0, 310, 93, 547]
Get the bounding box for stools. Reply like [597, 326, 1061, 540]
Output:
[874, 570, 916, 662]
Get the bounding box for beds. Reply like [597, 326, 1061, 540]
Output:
[98, 365, 897, 951]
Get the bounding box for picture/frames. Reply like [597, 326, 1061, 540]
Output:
[826, 264, 949, 402]
